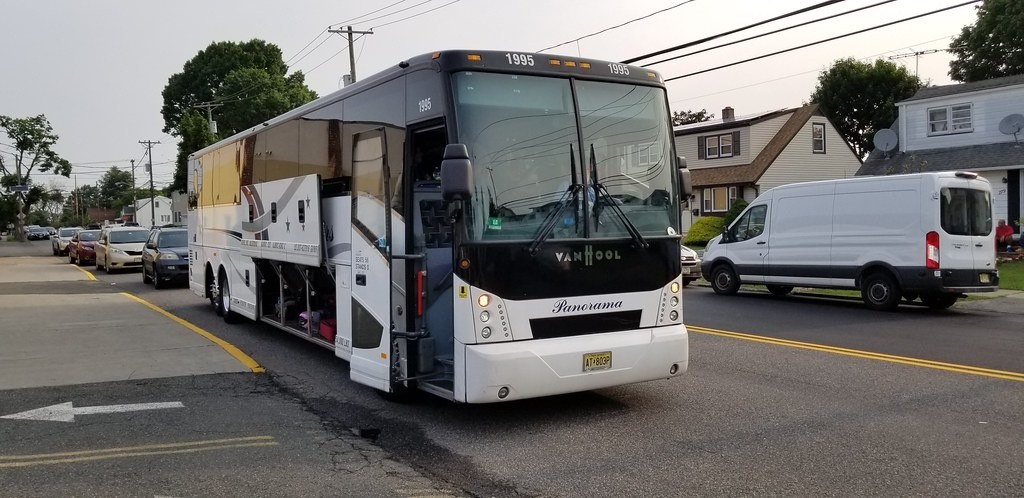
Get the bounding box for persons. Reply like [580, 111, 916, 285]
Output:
[994, 220, 1014, 252]
[553, 156, 600, 206]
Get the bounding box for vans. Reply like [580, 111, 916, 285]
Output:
[699, 170, 999, 312]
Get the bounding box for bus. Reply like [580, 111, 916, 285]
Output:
[187, 47, 695, 407]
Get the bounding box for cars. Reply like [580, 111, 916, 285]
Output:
[94, 224, 150, 275]
[17, 224, 85, 256]
[140, 224, 188, 290]
[68, 229, 101, 266]
[680, 244, 702, 286]
